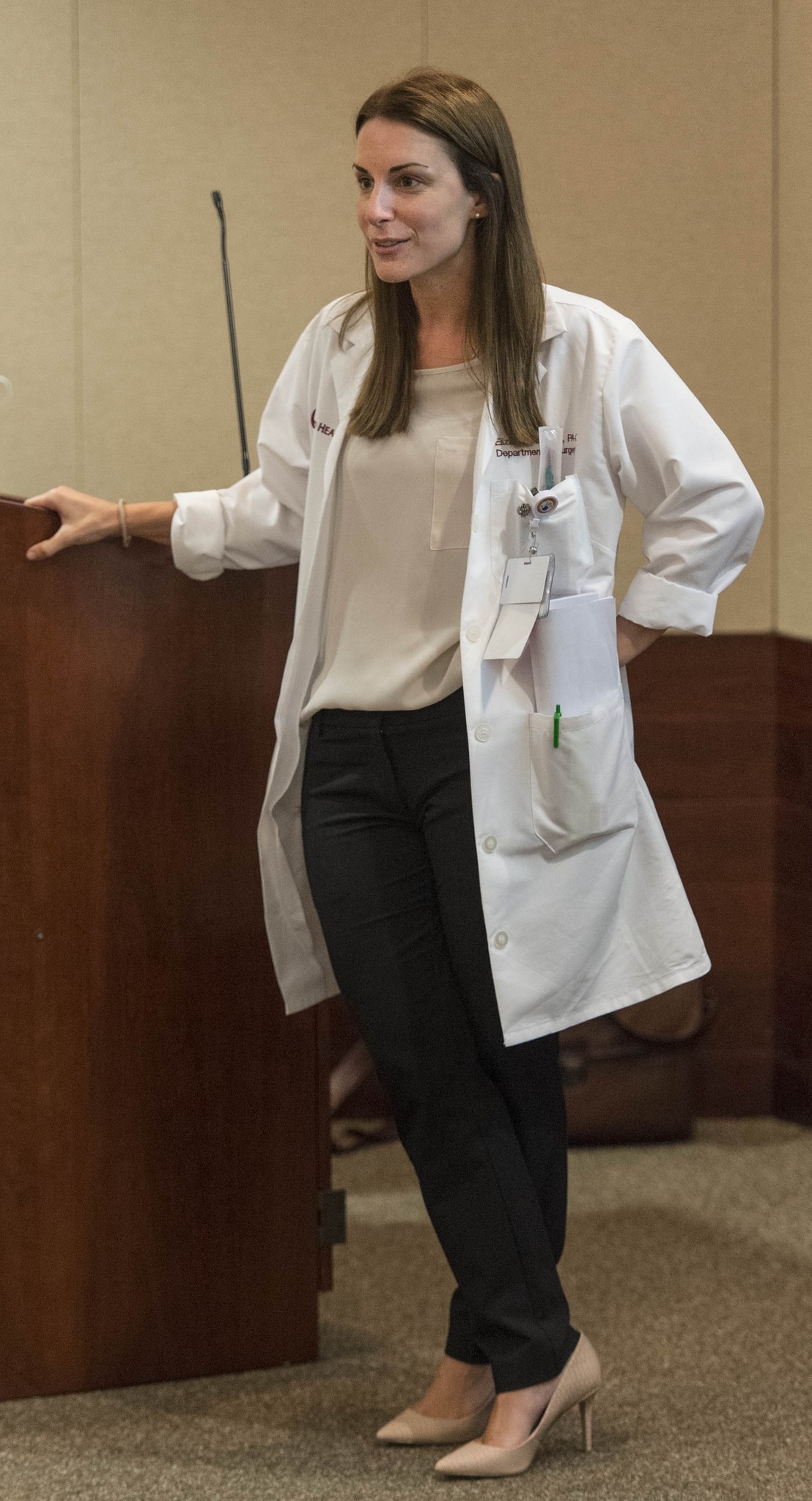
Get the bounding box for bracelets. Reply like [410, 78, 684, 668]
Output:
[117, 498, 132, 549]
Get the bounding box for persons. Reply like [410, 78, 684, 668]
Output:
[22, 66, 767, 1477]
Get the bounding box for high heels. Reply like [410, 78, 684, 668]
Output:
[377, 1388, 496, 1443]
[432, 1334, 601, 1474]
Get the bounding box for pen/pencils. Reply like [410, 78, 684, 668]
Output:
[553, 704, 562, 748]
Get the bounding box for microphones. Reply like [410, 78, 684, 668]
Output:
[212, 191, 251, 479]
